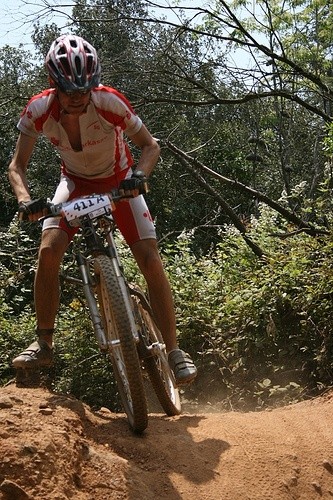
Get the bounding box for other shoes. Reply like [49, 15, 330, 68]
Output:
[168, 350, 197, 383]
[13, 341, 54, 367]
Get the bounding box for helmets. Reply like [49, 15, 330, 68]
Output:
[46, 34, 101, 91]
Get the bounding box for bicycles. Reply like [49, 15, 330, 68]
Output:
[23, 174, 185, 434]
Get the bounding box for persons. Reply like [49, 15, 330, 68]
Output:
[8, 34, 198, 389]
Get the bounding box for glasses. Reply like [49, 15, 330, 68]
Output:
[50, 75, 92, 96]
